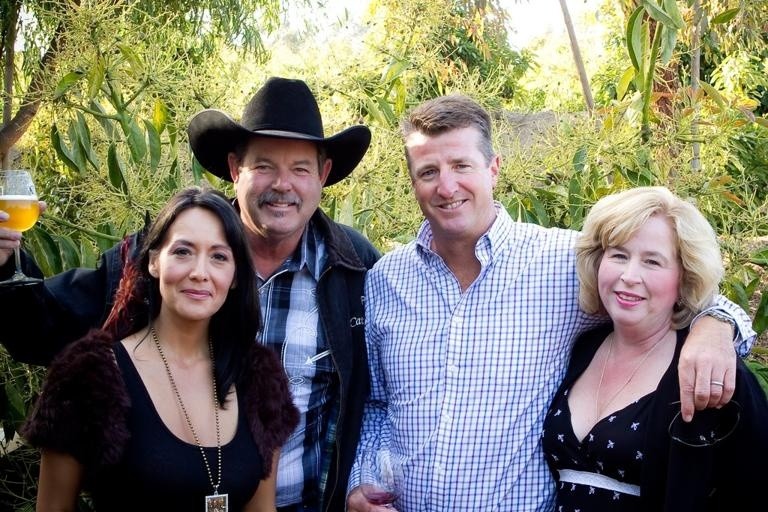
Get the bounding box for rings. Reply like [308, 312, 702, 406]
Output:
[711, 379, 725, 386]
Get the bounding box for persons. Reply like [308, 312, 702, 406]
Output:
[17, 185, 299, 512]
[536, 185, 768, 512]
[342, 97, 756, 510]
[0, 75, 383, 512]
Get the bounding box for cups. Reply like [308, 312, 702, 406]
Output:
[360, 448, 403, 506]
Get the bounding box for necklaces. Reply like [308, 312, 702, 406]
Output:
[596, 334, 668, 424]
[148, 322, 231, 512]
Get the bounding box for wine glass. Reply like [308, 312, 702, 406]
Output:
[0, 169, 43, 285]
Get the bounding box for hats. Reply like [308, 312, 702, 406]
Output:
[187, 76, 372, 187]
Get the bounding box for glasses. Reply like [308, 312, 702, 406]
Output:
[667, 398, 741, 450]
